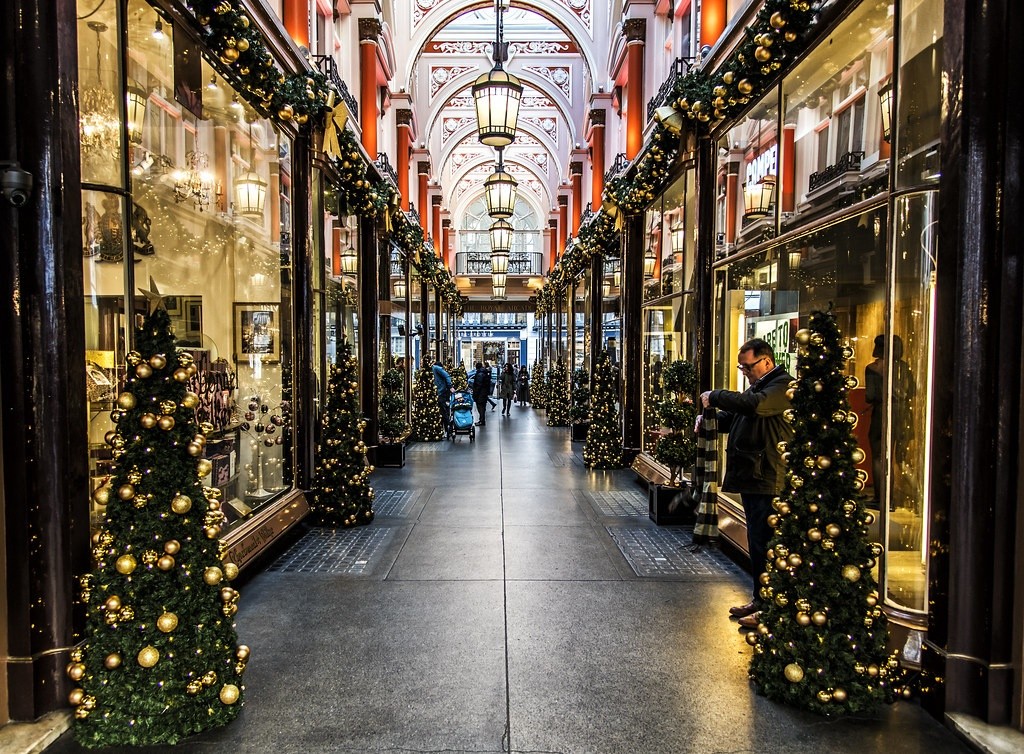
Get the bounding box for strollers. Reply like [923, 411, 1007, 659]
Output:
[446, 391, 475, 444]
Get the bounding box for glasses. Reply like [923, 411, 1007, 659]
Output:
[737, 357, 767, 374]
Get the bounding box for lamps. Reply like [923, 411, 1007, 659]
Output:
[492, 273, 507, 287]
[788, 252, 802, 270]
[484, 146, 517, 219]
[489, 251, 510, 274]
[643, 208, 657, 278]
[341, 216, 358, 275]
[743, 119, 775, 219]
[167, 117, 225, 211]
[394, 257, 405, 297]
[602, 260, 611, 297]
[490, 283, 508, 300]
[234, 115, 267, 217]
[472, 0, 524, 148]
[671, 220, 684, 254]
[489, 218, 515, 253]
[614, 258, 620, 287]
[76, 21, 145, 172]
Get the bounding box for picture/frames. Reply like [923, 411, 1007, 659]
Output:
[232, 301, 281, 363]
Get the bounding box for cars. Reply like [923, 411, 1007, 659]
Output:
[467, 367, 518, 394]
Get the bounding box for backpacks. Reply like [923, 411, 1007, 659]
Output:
[479, 371, 490, 388]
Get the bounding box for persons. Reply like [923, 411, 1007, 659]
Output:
[694, 339, 796, 629]
[394, 356, 415, 375]
[420, 358, 530, 437]
[863, 335, 923, 513]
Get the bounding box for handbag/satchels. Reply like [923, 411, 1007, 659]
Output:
[514, 394, 517, 403]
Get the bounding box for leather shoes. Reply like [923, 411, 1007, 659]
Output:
[729, 601, 757, 616]
[738, 610, 763, 628]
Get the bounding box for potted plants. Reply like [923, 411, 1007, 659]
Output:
[648, 359, 707, 524]
[377, 367, 407, 466]
[570, 368, 589, 441]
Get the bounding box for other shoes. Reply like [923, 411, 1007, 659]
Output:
[507, 411, 509, 416]
[491, 404, 496, 410]
[475, 421, 485, 426]
[502, 408, 506, 414]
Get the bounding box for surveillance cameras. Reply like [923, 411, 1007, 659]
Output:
[0, 168, 33, 206]
[397, 325, 406, 336]
[417, 324, 424, 337]
